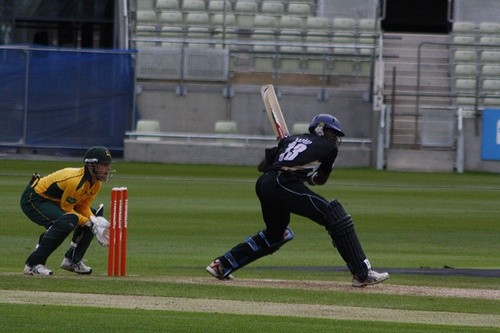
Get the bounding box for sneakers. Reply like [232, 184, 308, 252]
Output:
[352, 269, 389, 286]
[24, 264, 53, 275]
[206, 259, 233, 279]
[61, 257, 92, 274]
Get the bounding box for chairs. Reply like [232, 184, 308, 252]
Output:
[135, 120, 161, 143]
[214, 121, 238, 143]
[448, 20, 500, 119]
[129, 0, 383, 76]
[293, 122, 310, 135]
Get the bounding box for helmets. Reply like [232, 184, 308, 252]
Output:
[308, 113, 345, 149]
[83, 145, 116, 183]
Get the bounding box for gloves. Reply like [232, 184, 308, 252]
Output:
[86, 215, 109, 246]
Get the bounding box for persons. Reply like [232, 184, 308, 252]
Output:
[20, 147, 112, 275]
[206, 115, 389, 286]
